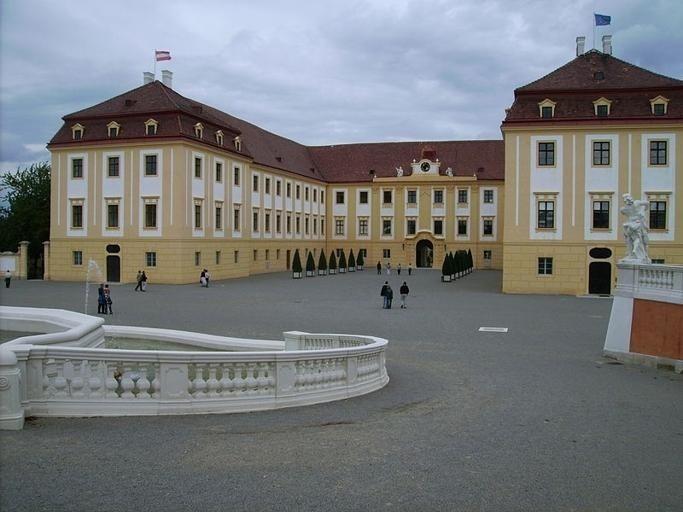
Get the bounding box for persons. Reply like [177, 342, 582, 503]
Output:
[400, 281, 409, 308]
[4, 269, 12, 288]
[380, 281, 390, 309]
[377, 261, 413, 275]
[141, 271, 147, 292]
[387, 288, 393, 309]
[200, 270, 207, 287]
[205, 270, 210, 287]
[135, 271, 142, 291]
[620, 193, 651, 264]
[103, 284, 113, 314]
[98, 284, 105, 315]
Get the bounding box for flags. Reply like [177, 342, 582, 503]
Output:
[594, 13, 611, 26]
[155, 49, 171, 61]
